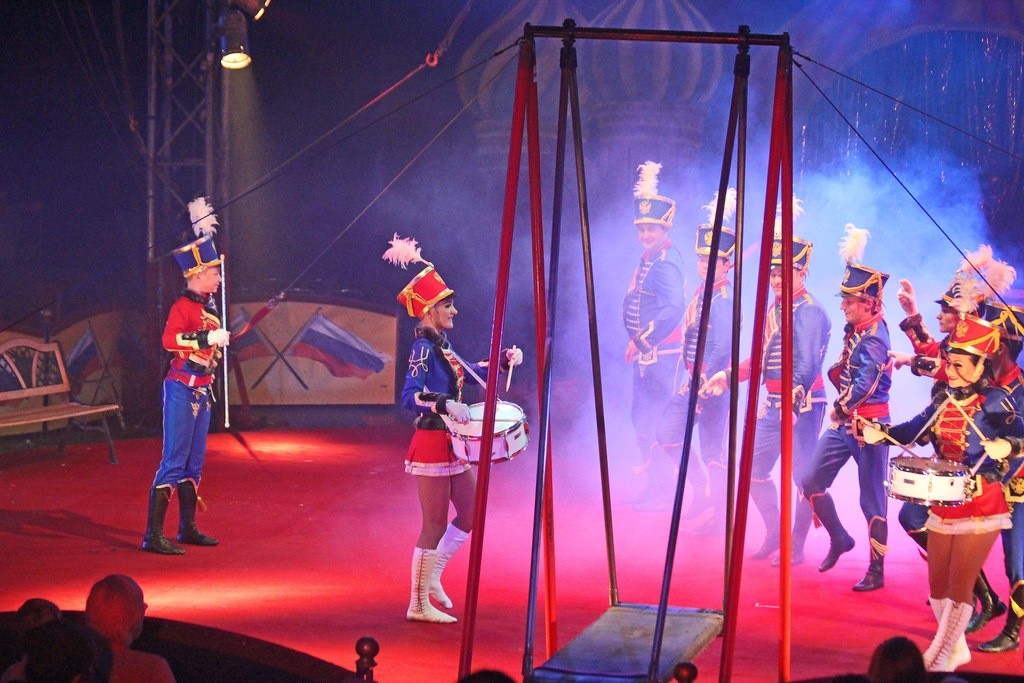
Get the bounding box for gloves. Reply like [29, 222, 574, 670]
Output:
[444, 399, 472, 425]
[208, 329, 231, 347]
[506, 348, 523, 366]
[863, 423, 886, 445]
[979, 435, 1012, 459]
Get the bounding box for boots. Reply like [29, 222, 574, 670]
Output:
[749, 478, 780, 559]
[927, 595, 971, 672]
[641, 441, 728, 534]
[177, 476, 219, 545]
[908, 527, 927, 554]
[802, 488, 855, 571]
[923, 598, 973, 671]
[429, 522, 469, 608]
[143, 483, 185, 554]
[967, 569, 1007, 632]
[406, 547, 457, 623]
[771, 490, 812, 566]
[978, 580, 1024, 652]
[853, 516, 887, 591]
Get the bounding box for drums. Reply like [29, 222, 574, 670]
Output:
[448, 400, 529, 466]
[885, 455, 970, 505]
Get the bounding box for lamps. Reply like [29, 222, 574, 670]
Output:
[219, 0, 271, 70]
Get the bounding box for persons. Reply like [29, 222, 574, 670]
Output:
[142, 234, 231, 555]
[867, 636, 927, 683]
[397, 266, 522, 623]
[623, 195, 1024, 670]
[459, 670, 514, 683]
[0, 574, 175, 683]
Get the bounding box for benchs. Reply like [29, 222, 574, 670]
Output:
[0, 339, 119, 465]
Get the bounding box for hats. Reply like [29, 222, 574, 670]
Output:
[172, 197, 222, 278]
[835, 223, 890, 301]
[694, 187, 737, 258]
[986, 258, 1024, 341]
[770, 192, 813, 270]
[935, 244, 993, 318]
[946, 277, 1000, 361]
[633, 160, 675, 225]
[382, 233, 454, 319]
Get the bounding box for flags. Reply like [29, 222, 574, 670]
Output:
[66, 329, 102, 397]
[230, 313, 272, 371]
[113, 323, 148, 373]
[290, 314, 392, 380]
